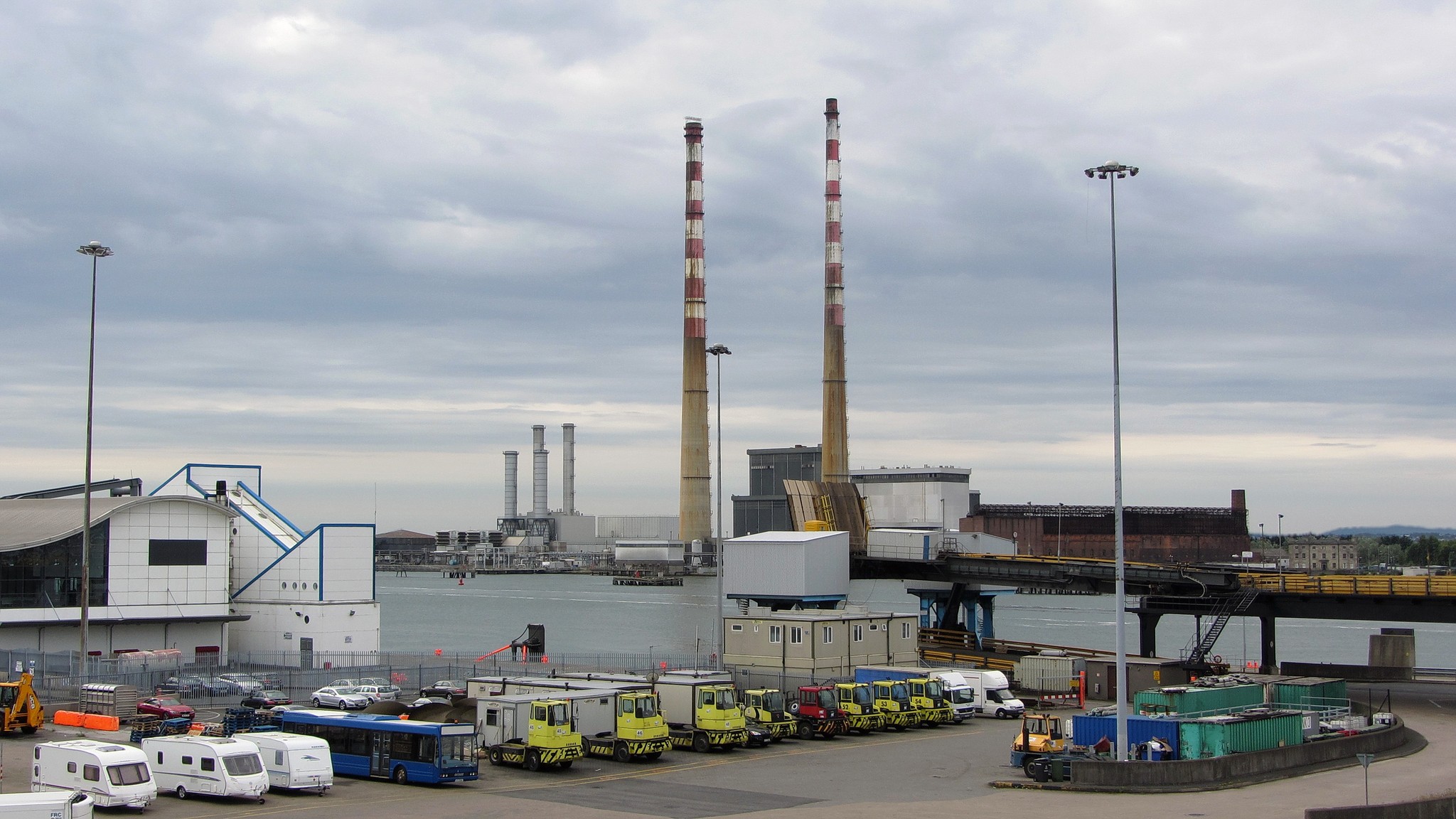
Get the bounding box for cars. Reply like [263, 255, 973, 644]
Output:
[240, 690, 293, 710]
[407, 696, 453, 708]
[251, 672, 284, 691]
[151, 673, 240, 699]
[419, 679, 467, 700]
[327, 677, 402, 705]
[268, 705, 307, 716]
[215, 673, 263, 695]
[137, 696, 196, 721]
[309, 687, 370, 710]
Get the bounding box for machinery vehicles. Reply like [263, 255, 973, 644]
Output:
[0, 672, 46, 738]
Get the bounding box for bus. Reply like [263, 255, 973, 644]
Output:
[282, 710, 486, 786]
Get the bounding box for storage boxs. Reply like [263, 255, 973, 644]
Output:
[1018, 655, 1346, 759]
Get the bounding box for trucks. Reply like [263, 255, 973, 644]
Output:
[30, 739, 157, 815]
[139, 732, 270, 804]
[445, 662, 1182, 784]
[230, 731, 334, 797]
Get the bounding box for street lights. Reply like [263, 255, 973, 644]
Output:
[1057, 502, 1064, 561]
[940, 498, 945, 551]
[1027, 501, 1031, 555]
[1084, 162, 1140, 764]
[76, 240, 115, 711]
[1278, 514, 1284, 576]
[1259, 523, 1264, 569]
[703, 342, 734, 671]
[862, 496, 869, 547]
[1357, 557, 1362, 575]
